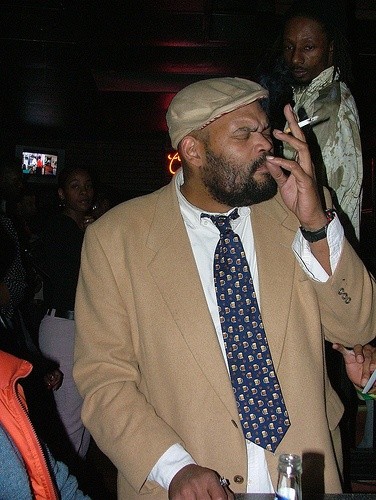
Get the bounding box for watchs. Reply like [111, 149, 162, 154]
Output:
[299, 208, 334, 243]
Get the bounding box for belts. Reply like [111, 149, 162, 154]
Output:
[47, 309, 75, 320]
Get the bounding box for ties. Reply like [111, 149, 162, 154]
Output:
[200, 208, 291, 454]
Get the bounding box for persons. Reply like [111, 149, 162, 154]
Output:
[28, 155, 53, 175]
[332, 343, 376, 406]
[72, 77, 376, 500]
[39, 165, 117, 462]
[13, 189, 47, 305]
[0, 212, 63, 440]
[281, 10, 364, 251]
[0, 230, 91, 500]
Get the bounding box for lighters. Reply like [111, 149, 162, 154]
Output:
[219, 475, 230, 487]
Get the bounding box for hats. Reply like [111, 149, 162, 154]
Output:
[165, 76, 269, 151]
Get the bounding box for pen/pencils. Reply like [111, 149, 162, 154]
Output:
[220, 477, 228, 488]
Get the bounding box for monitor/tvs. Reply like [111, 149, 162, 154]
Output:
[14, 145, 67, 186]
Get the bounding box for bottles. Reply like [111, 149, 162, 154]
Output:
[274, 454, 303, 500]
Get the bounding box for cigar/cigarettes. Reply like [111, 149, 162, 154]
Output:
[361, 370, 376, 394]
